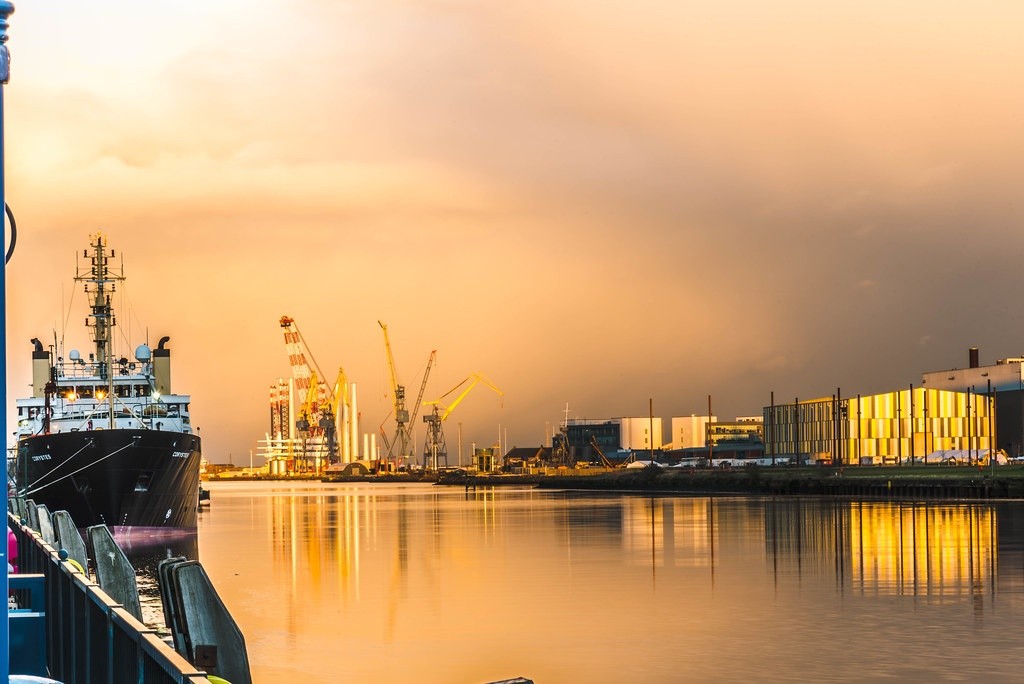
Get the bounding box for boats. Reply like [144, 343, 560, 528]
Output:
[11, 233, 214, 551]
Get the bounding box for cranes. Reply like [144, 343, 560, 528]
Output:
[265, 314, 353, 475]
[372, 320, 507, 474]
